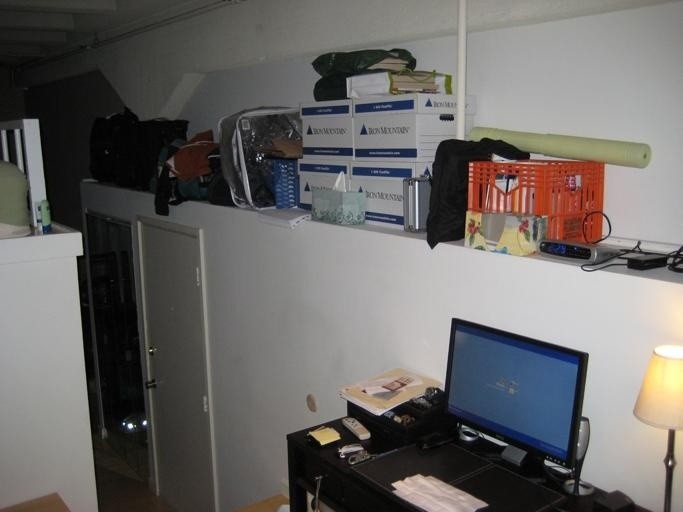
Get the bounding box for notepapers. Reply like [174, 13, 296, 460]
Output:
[308, 427, 341, 446]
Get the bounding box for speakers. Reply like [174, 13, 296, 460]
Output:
[563, 416, 594, 496]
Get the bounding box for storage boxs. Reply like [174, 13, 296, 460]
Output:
[295, 93, 476, 232]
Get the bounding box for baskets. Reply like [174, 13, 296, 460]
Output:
[467, 160, 605, 247]
[274, 160, 301, 208]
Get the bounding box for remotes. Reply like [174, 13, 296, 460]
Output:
[342, 416, 371, 440]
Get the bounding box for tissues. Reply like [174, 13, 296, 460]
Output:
[311, 172, 366, 224]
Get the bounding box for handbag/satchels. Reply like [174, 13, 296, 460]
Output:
[426, 137, 530, 249]
[219, 106, 302, 209]
[90, 118, 186, 192]
[345, 70, 453, 99]
[174, 140, 216, 201]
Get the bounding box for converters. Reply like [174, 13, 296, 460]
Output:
[594, 490, 635, 512]
[625, 253, 670, 271]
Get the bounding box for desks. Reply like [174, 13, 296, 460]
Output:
[285, 417, 653, 511]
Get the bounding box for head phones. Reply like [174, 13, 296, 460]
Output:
[411, 387, 444, 412]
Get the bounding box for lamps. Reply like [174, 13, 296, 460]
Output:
[633, 343, 683, 511]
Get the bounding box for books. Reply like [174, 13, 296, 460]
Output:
[338, 367, 444, 417]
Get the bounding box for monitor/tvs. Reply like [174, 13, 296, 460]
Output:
[440, 318, 589, 469]
